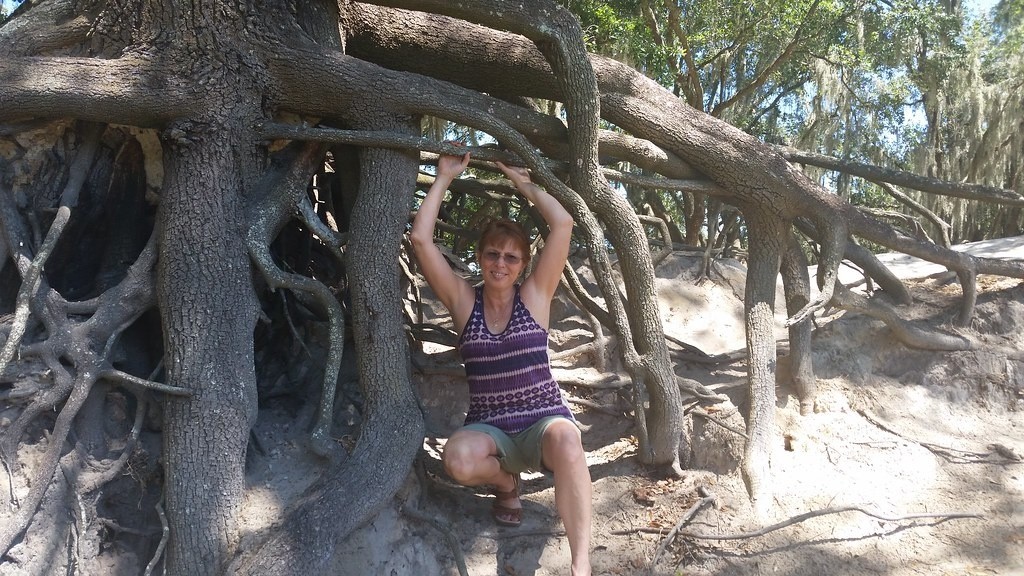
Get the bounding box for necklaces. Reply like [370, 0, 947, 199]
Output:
[485, 290, 515, 327]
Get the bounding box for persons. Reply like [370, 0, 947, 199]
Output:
[410, 140, 592, 576]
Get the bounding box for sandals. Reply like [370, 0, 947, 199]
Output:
[493, 472, 522, 526]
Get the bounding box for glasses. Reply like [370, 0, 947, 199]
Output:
[481, 249, 524, 264]
